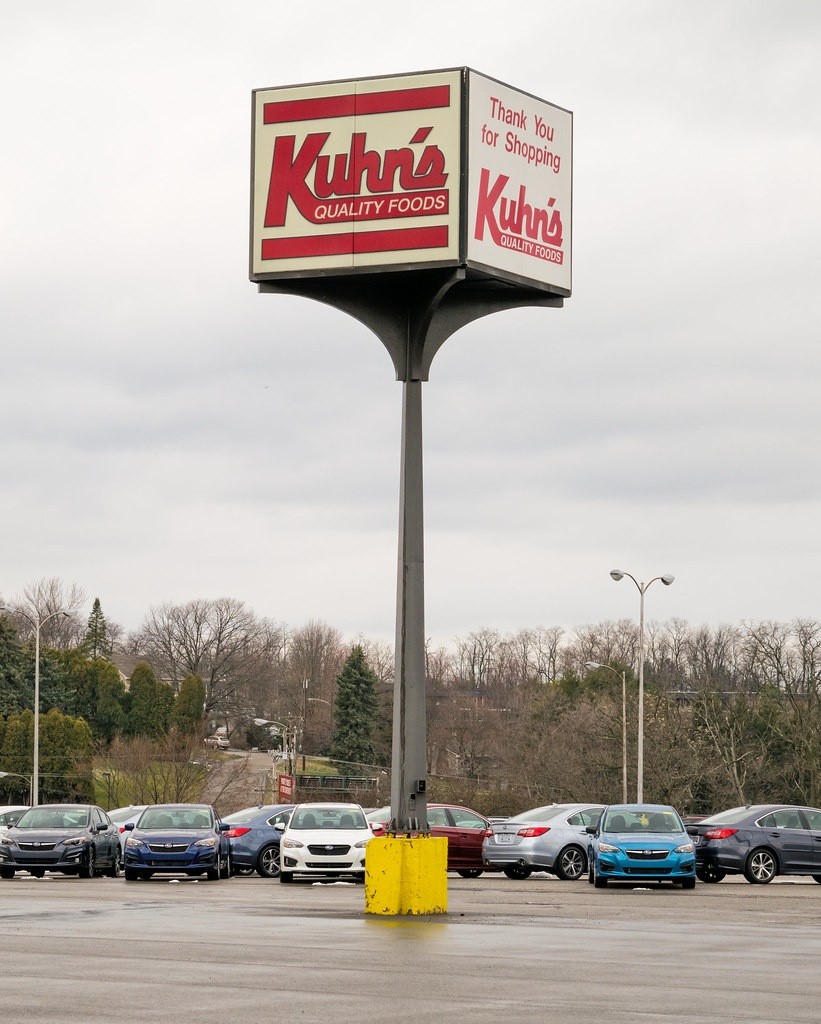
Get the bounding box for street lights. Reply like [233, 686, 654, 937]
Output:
[102, 771, 111, 809]
[252, 719, 296, 777]
[0, 605, 79, 809]
[585, 662, 628, 804]
[609, 568, 677, 802]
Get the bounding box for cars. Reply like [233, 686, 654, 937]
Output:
[479, 802, 645, 880]
[585, 803, 699, 888]
[274, 801, 383, 883]
[0, 806, 76, 845]
[203, 735, 231, 751]
[217, 803, 343, 878]
[680, 813, 715, 834]
[456, 814, 512, 828]
[363, 802, 504, 878]
[101, 805, 164, 870]
[684, 804, 821, 886]
[0, 804, 122, 878]
[120, 803, 232, 880]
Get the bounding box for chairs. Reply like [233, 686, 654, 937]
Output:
[606, 815, 629, 831]
[68, 816, 94, 827]
[649, 813, 672, 831]
[766, 817, 777, 827]
[188, 815, 207, 828]
[340, 814, 356, 828]
[784, 815, 801, 828]
[302, 814, 321, 828]
[151, 814, 172, 826]
